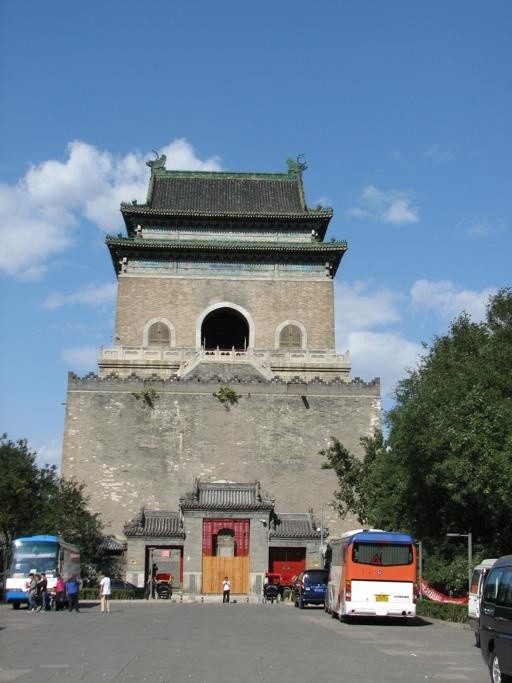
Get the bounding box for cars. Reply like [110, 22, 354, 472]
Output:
[102, 580, 145, 596]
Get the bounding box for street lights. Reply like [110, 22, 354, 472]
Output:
[319, 499, 337, 570]
[445, 531, 473, 595]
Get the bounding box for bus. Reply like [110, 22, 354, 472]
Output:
[5, 533, 82, 610]
[324, 528, 418, 623]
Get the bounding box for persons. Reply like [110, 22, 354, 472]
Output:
[24, 570, 233, 613]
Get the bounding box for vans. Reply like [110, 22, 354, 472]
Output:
[480, 554, 512, 683]
[468, 557, 499, 648]
[292, 569, 328, 609]
